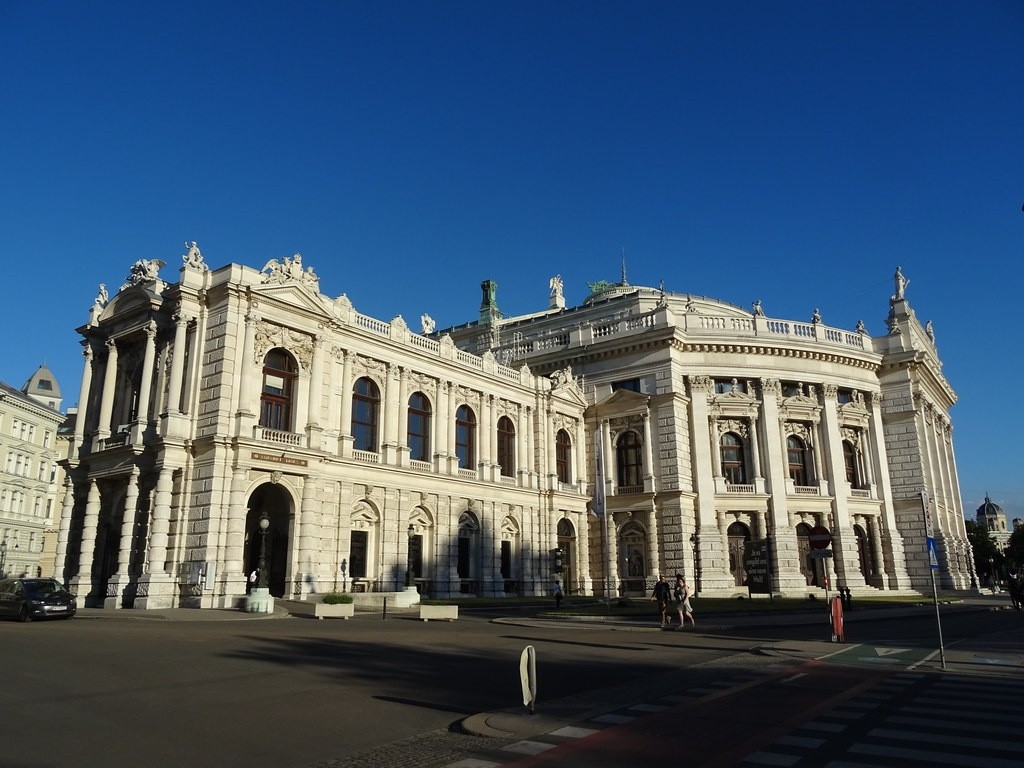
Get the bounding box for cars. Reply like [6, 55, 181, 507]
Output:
[0, 578, 77, 622]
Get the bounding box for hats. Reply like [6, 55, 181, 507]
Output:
[659, 574, 666, 576]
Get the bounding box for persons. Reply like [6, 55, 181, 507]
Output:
[673, 574, 695, 629]
[754, 300, 766, 317]
[657, 290, 669, 309]
[685, 295, 696, 312]
[811, 308, 822, 324]
[182, 240, 200, 268]
[554, 580, 563, 608]
[651, 574, 671, 628]
[927, 320, 936, 345]
[551, 274, 563, 295]
[96, 283, 109, 301]
[895, 266, 910, 300]
[260, 253, 329, 305]
[856, 319, 869, 336]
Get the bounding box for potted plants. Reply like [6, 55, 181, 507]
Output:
[315, 593, 354, 620]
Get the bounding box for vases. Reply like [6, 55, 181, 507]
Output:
[420, 605, 458, 621]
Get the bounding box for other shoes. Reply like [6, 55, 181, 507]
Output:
[692, 623, 695, 628]
[660, 621, 665, 628]
[678, 625, 684, 628]
[666, 615, 671, 624]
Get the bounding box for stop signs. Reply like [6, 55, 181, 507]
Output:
[808, 526, 831, 549]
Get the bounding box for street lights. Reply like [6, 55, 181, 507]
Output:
[689, 533, 702, 600]
[245, 512, 274, 614]
